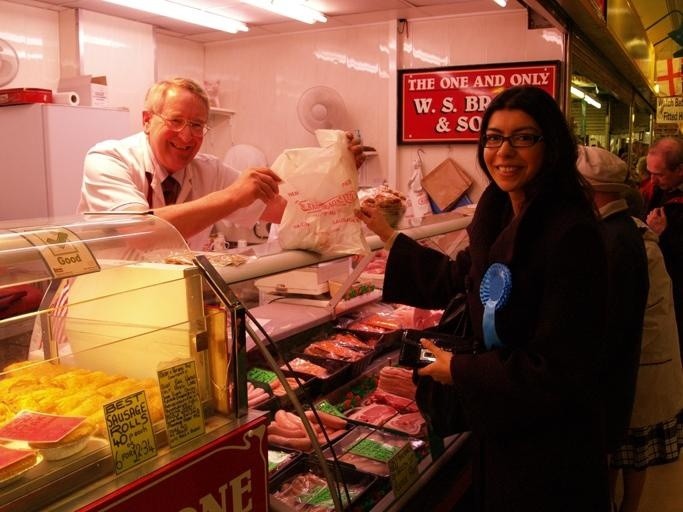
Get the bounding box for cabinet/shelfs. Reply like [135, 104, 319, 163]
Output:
[210, 216, 476, 511]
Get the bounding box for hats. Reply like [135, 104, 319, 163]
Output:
[576, 143, 632, 194]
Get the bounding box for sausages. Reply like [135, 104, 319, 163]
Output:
[246, 376, 347, 452]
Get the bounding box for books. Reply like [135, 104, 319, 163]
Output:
[421, 158, 476, 215]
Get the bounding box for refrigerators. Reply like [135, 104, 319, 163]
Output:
[1, 104, 131, 219]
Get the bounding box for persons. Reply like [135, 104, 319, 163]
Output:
[354, 84, 605, 511]
[606, 182, 682, 511]
[576, 142, 650, 511]
[76, 78, 367, 263]
[612, 136, 683, 361]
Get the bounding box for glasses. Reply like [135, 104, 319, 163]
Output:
[479, 132, 545, 149]
[149, 109, 210, 138]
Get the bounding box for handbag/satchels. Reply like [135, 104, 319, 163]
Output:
[410, 289, 548, 440]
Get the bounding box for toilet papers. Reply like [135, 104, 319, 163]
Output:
[53, 92, 79, 108]
